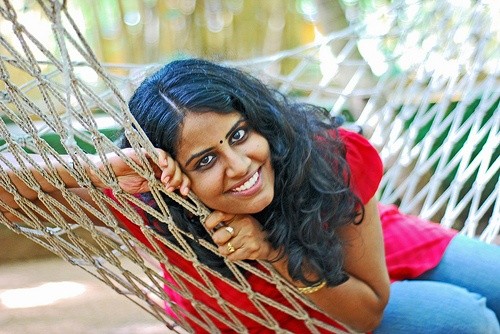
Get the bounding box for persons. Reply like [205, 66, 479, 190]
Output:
[0, 58, 500, 334]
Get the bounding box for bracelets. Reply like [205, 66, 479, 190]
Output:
[298, 279, 327, 294]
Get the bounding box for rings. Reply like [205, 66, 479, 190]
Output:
[226, 227, 234, 235]
[228, 243, 235, 253]
